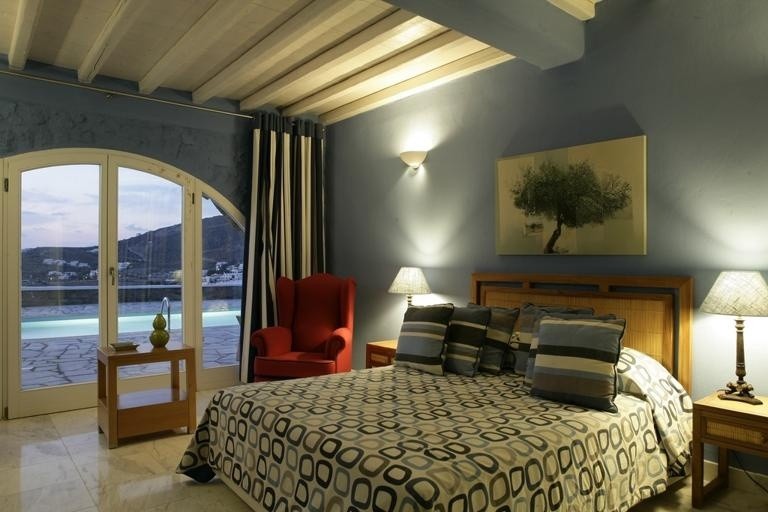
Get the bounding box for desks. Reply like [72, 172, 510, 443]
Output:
[691, 391, 768, 510]
[364, 338, 398, 369]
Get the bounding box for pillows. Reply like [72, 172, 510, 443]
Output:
[513, 301, 595, 377]
[466, 301, 520, 377]
[394, 302, 455, 376]
[442, 305, 494, 379]
[528, 314, 629, 413]
[520, 310, 617, 394]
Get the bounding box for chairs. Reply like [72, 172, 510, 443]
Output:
[250, 269, 358, 384]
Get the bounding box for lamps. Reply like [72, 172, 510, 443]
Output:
[396, 148, 430, 171]
[695, 269, 768, 404]
[388, 265, 435, 314]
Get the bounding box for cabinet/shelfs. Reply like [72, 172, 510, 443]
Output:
[95, 340, 198, 451]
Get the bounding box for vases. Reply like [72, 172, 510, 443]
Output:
[149, 312, 170, 347]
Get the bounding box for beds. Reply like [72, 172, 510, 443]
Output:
[174, 269, 696, 511]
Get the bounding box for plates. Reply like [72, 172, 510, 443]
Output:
[109, 341, 139, 351]
